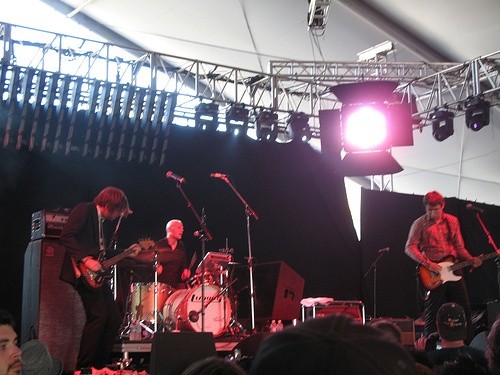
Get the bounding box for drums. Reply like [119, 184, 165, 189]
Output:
[129, 280, 176, 325]
[162, 283, 232, 337]
[184, 273, 217, 290]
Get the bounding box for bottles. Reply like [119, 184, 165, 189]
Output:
[129, 320, 136, 340]
[136, 319, 141, 341]
[270, 320, 277, 333]
[276, 319, 284, 333]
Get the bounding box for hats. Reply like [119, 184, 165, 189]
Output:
[249, 312, 402, 375]
[20, 339, 63, 375]
[437, 303, 468, 342]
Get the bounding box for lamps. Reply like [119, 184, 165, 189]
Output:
[254, 111, 280, 143]
[465, 98, 490, 130]
[315, 78, 421, 176]
[225, 107, 251, 137]
[430, 109, 455, 141]
[287, 112, 313, 145]
[195, 102, 219, 130]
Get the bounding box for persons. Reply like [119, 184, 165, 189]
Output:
[20, 339, 64, 375]
[155, 219, 191, 289]
[404, 191, 484, 353]
[180, 302, 500, 375]
[59, 186, 141, 375]
[0, 310, 23, 375]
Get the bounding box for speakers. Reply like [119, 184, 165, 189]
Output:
[228, 261, 305, 321]
[21, 239, 86, 373]
[150, 332, 217, 375]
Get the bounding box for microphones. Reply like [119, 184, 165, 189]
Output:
[466, 203, 484, 213]
[190, 314, 199, 322]
[194, 229, 203, 236]
[166, 171, 185, 183]
[210, 173, 229, 178]
[378, 247, 390, 253]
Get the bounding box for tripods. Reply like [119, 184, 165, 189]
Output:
[120, 251, 165, 343]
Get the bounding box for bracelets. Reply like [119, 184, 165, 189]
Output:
[84, 257, 88, 264]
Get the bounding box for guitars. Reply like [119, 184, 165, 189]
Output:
[416, 249, 500, 291]
[79, 237, 155, 289]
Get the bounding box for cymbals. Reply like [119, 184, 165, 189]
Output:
[134, 248, 185, 263]
